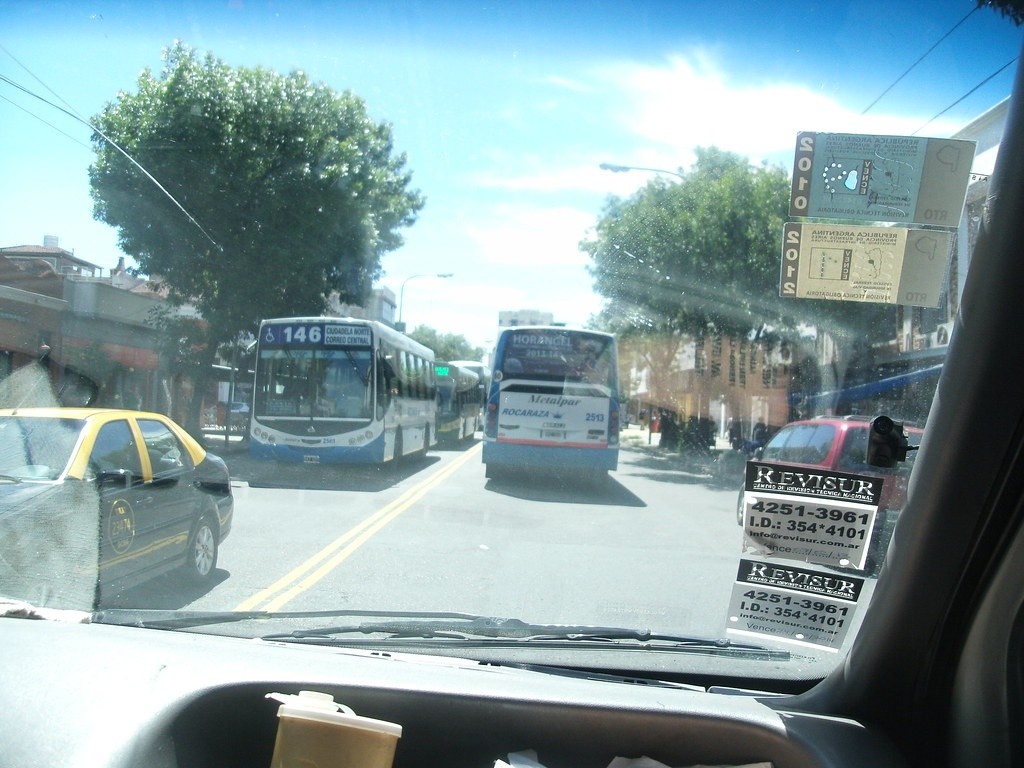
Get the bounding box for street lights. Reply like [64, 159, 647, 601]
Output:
[398, 271, 454, 321]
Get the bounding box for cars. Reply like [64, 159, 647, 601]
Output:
[0, 407, 236, 613]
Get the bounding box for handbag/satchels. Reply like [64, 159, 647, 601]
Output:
[725, 430, 730, 439]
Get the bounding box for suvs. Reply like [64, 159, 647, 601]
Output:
[231, 401, 249, 416]
[736, 415, 924, 570]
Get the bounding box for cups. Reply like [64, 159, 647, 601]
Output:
[264, 689, 403, 768]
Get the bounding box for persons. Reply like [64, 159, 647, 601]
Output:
[639, 409, 648, 430]
[726, 416, 733, 445]
[752, 417, 766, 440]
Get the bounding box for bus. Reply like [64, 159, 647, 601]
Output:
[435, 360, 483, 446]
[481, 326, 619, 488]
[239, 318, 436, 473]
